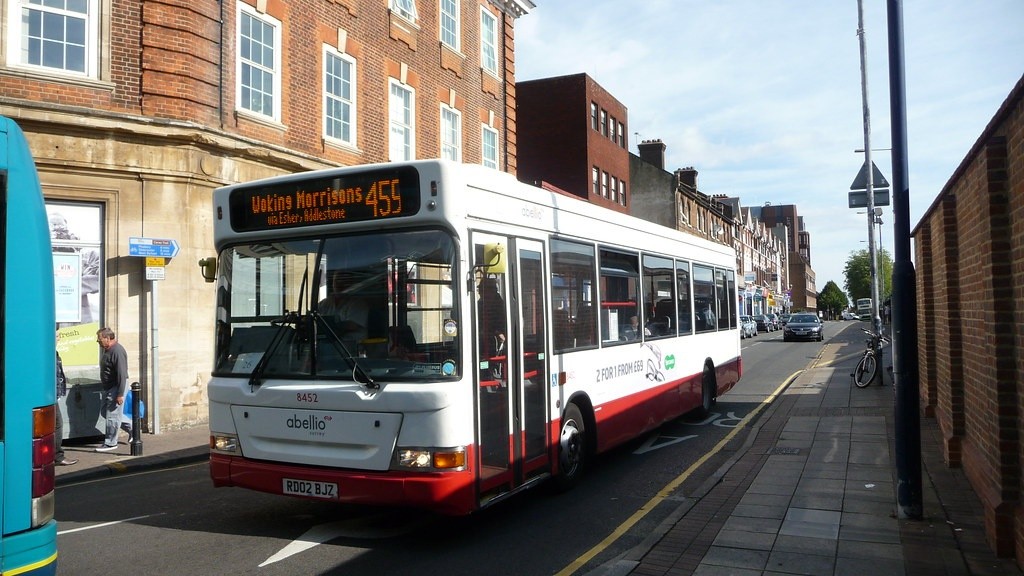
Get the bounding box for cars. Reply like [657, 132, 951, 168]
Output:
[779, 312, 825, 342]
[739, 312, 790, 339]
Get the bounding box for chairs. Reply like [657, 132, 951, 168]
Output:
[552, 297, 729, 350]
[389, 324, 452, 364]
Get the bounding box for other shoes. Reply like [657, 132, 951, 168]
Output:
[128, 429, 142, 443]
[95, 444, 118, 452]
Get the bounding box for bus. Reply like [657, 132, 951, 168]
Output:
[199, 158, 743, 517]
[857, 298, 872, 320]
[0, 115, 58, 576]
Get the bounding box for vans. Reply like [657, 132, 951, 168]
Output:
[843, 313, 859, 321]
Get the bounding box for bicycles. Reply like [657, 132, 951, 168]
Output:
[851, 328, 890, 388]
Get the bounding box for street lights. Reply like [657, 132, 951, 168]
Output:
[857, 212, 884, 306]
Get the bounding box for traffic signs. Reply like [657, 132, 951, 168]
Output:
[129, 237, 180, 259]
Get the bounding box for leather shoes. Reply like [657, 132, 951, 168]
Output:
[58, 456, 79, 465]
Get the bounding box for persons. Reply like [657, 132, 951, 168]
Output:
[95, 328, 133, 452]
[55, 323, 79, 466]
[478, 277, 505, 332]
[884, 305, 891, 325]
[620, 315, 651, 340]
[318, 270, 368, 342]
